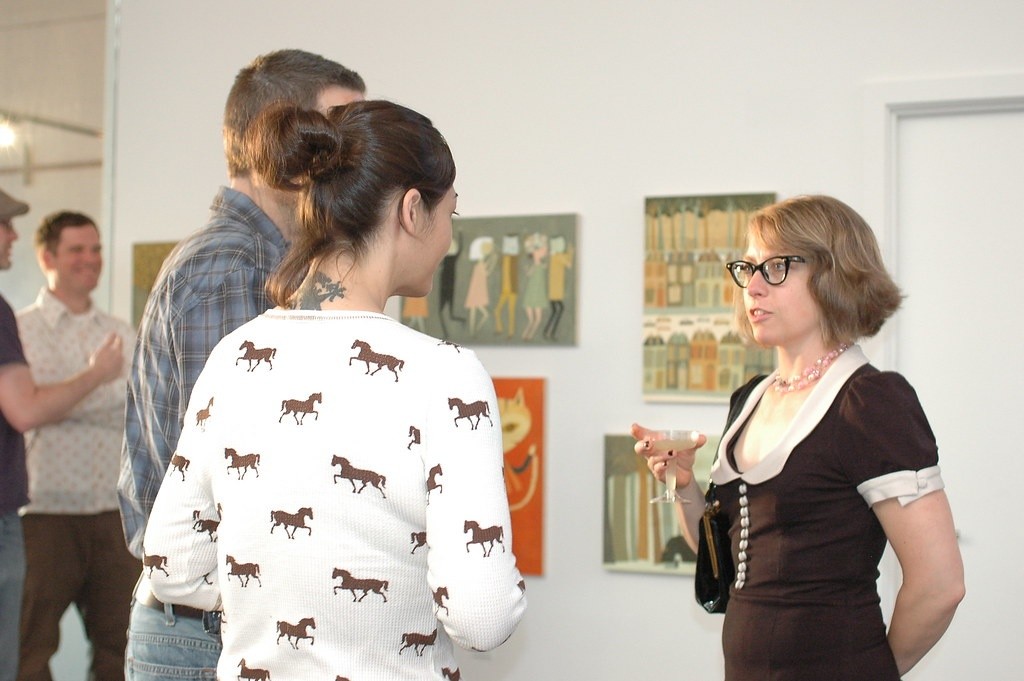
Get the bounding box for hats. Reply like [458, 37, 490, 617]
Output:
[0, 191, 29, 218]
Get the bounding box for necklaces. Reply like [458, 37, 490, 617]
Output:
[774, 338, 853, 394]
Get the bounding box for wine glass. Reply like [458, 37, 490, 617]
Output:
[640, 409, 699, 504]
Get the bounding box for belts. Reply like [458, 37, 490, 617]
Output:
[134, 572, 222, 620]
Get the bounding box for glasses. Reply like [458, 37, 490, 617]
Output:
[0, 217, 13, 231]
[726, 256, 806, 288]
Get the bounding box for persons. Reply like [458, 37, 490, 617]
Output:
[632, 196, 966, 681]
[0, 48, 527, 681]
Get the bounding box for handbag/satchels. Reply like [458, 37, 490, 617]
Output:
[694, 500, 735, 615]
